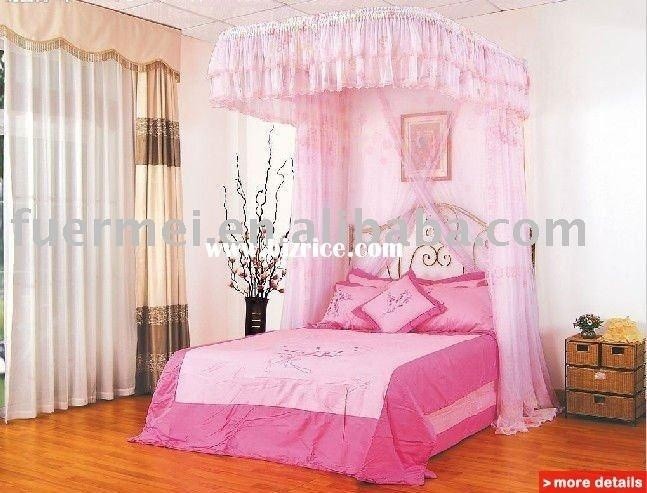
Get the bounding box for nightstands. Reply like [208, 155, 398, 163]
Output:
[564, 332, 646, 427]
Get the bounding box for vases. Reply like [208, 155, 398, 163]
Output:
[580, 329, 597, 340]
[244, 297, 269, 336]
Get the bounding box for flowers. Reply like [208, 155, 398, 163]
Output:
[219, 123, 295, 297]
[573, 314, 604, 329]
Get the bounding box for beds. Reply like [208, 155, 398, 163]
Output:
[126, 201, 536, 487]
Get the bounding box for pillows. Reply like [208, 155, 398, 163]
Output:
[317, 280, 383, 331]
[346, 267, 394, 291]
[409, 286, 493, 334]
[416, 271, 488, 288]
[350, 268, 447, 334]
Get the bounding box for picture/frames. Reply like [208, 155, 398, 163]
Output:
[400, 110, 452, 183]
